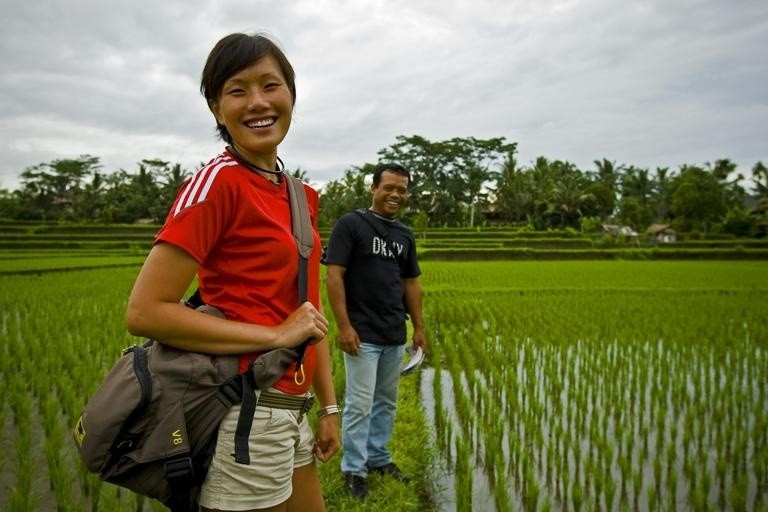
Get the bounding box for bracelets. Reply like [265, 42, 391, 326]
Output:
[316, 405, 341, 420]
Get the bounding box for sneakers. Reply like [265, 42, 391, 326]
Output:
[343, 475, 364, 504]
[374, 463, 410, 483]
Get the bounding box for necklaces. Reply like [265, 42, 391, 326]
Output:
[229, 143, 285, 174]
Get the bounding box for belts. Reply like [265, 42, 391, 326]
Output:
[256, 390, 315, 414]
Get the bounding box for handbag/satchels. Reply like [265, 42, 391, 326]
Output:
[401, 291, 410, 314]
[72, 304, 299, 511]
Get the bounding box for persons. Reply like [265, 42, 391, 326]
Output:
[126, 33, 341, 512]
[323, 163, 436, 499]
